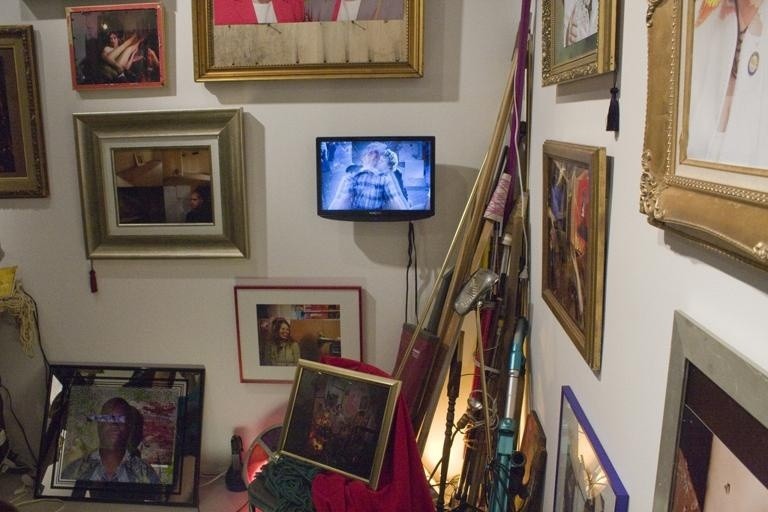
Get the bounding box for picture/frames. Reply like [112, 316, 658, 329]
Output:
[652, 308, 768, 512]
[33, 362, 206, 509]
[277, 357, 403, 492]
[0, 24, 51, 199]
[134, 152, 146, 166]
[541, 0, 618, 88]
[63, 2, 166, 91]
[70, 106, 253, 261]
[639, 0, 768, 275]
[233, 285, 362, 385]
[190, 0, 424, 83]
[553, 384, 630, 512]
[541, 139, 607, 379]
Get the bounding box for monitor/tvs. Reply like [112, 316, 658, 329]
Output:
[316, 136, 437, 224]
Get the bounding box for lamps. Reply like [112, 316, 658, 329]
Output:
[456, 397, 484, 430]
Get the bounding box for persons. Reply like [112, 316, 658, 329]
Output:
[261, 317, 301, 365]
[101, 31, 145, 74]
[186, 190, 211, 223]
[60, 398, 160, 483]
[328, 142, 414, 210]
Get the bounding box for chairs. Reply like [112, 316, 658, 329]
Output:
[245, 354, 436, 512]
